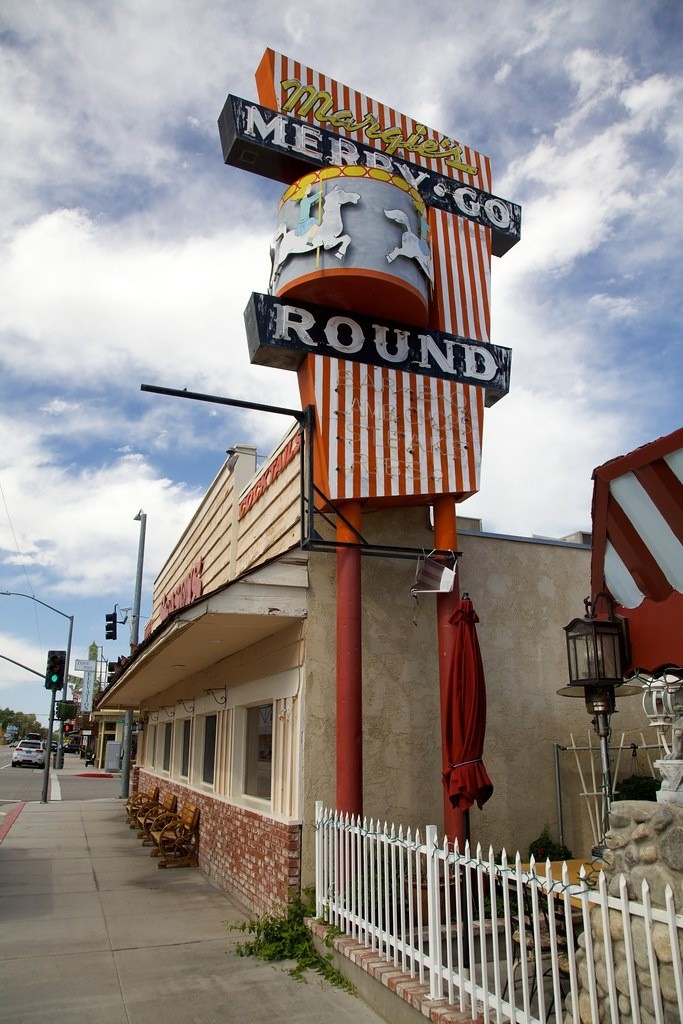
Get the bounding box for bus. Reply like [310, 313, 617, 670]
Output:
[25, 733, 41, 740]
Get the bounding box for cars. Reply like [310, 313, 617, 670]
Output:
[54, 744, 80, 754]
[10, 743, 16, 748]
[51, 741, 57, 750]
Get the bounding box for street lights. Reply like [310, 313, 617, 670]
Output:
[0, 591, 73, 804]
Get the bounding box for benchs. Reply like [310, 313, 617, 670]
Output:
[130, 792, 176, 839]
[123, 786, 158, 824]
[141, 802, 200, 869]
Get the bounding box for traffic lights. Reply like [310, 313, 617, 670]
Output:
[62, 723, 73, 732]
[105, 612, 117, 641]
[45, 650, 66, 691]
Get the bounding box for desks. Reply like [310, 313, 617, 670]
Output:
[494, 858, 611, 972]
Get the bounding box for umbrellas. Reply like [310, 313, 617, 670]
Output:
[448, 591, 493, 972]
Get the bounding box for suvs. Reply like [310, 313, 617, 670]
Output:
[12, 740, 46, 768]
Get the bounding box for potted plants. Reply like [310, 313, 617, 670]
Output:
[399, 855, 462, 927]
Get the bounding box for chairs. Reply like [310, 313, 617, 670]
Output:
[495, 873, 578, 1024]
[86, 754, 96, 767]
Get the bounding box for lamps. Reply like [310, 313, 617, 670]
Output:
[137, 717, 144, 730]
[132, 720, 136, 732]
